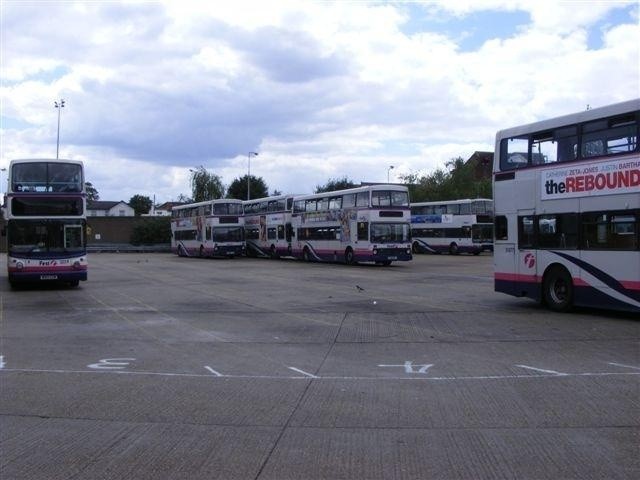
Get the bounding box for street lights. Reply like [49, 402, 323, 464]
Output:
[248, 152, 258, 199]
[388, 165, 394, 184]
[55, 99, 65, 159]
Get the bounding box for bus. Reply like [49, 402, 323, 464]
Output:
[408, 199, 493, 255]
[3, 157, 89, 290]
[492, 98, 639, 320]
[168, 185, 414, 268]
